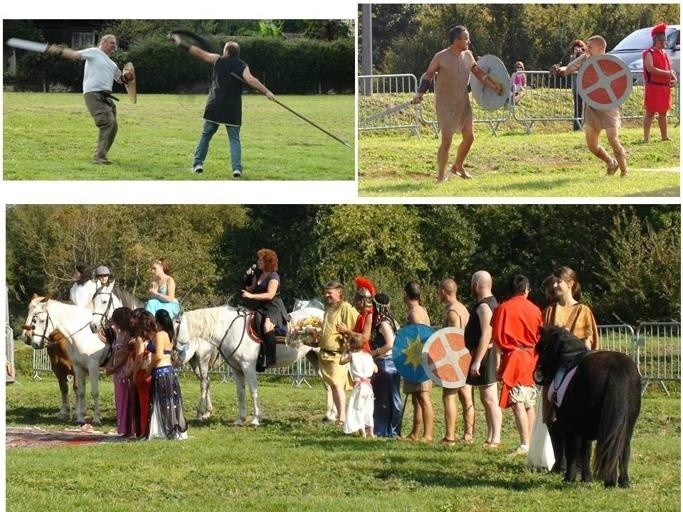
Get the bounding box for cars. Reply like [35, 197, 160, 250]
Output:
[605, 25, 680, 87]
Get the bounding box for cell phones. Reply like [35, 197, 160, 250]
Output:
[576, 52, 582, 57]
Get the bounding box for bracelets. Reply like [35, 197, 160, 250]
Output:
[178, 41, 192, 54]
[418, 75, 430, 95]
[56, 47, 64, 55]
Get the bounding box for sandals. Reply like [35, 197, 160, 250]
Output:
[604, 160, 618, 175]
[334, 420, 345, 428]
[404, 431, 530, 455]
[620, 168, 628, 178]
[450, 165, 472, 179]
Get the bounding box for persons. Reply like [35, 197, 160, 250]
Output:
[507, 61, 527, 105]
[169, 34, 276, 178]
[413, 24, 504, 184]
[72, 261, 190, 445]
[46, 33, 134, 164]
[240, 249, 292, 374]
[557, 36, 633, 179]
[643, 23, 678, 144]
[551, 40, 587, 130]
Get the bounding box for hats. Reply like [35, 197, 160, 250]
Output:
[652, 32, 668, 45]
[354, 279, 373, 306]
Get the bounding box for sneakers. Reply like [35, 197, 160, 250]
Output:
[233, 170, 242, 177]
[93, 154, 110, 164]
[193, 166, 203, 173]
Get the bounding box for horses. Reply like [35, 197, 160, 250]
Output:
[532, 324, 642, 489]
[21, 291, 78, 421]
[31, 297, 120, 426]
[170, 306, 339, 428]
[89, 278, 216, 424]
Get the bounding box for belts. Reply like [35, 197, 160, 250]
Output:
[503, 347, 532, 353]
[469, 343, 493, 356]
[94, 91, 119, 102]
[646, 80, 670, 86]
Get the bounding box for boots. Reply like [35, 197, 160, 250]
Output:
[264, 331, 278, 367]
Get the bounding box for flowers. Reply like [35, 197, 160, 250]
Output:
[285, 317, 323, 350]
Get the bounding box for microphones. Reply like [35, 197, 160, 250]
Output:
[244, 264, 257, 278]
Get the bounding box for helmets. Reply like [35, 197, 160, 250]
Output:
[95, 266, 110, 276]
